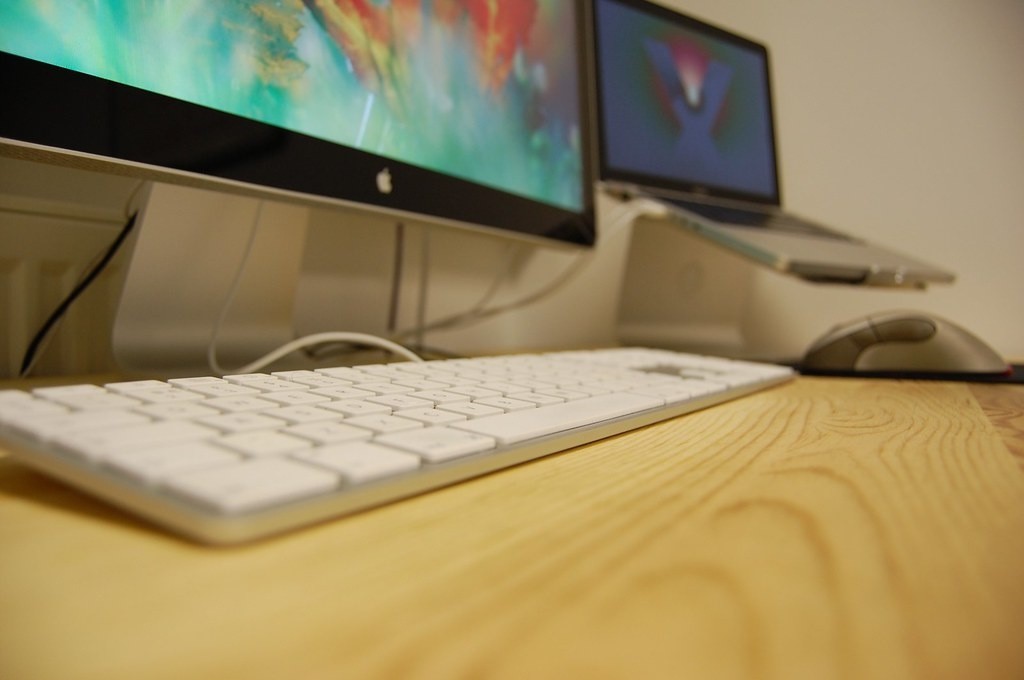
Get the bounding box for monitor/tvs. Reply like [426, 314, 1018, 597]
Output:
[0, 0, 597, 378]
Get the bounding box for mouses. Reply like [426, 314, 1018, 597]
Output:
[799, 308, 1010, 376]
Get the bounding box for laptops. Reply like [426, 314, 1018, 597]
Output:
[595, 0, 955, 283]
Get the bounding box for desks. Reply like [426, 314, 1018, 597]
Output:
[0, 338, 1024, 680]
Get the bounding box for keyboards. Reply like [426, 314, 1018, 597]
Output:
[0, 349, 795, 547]
